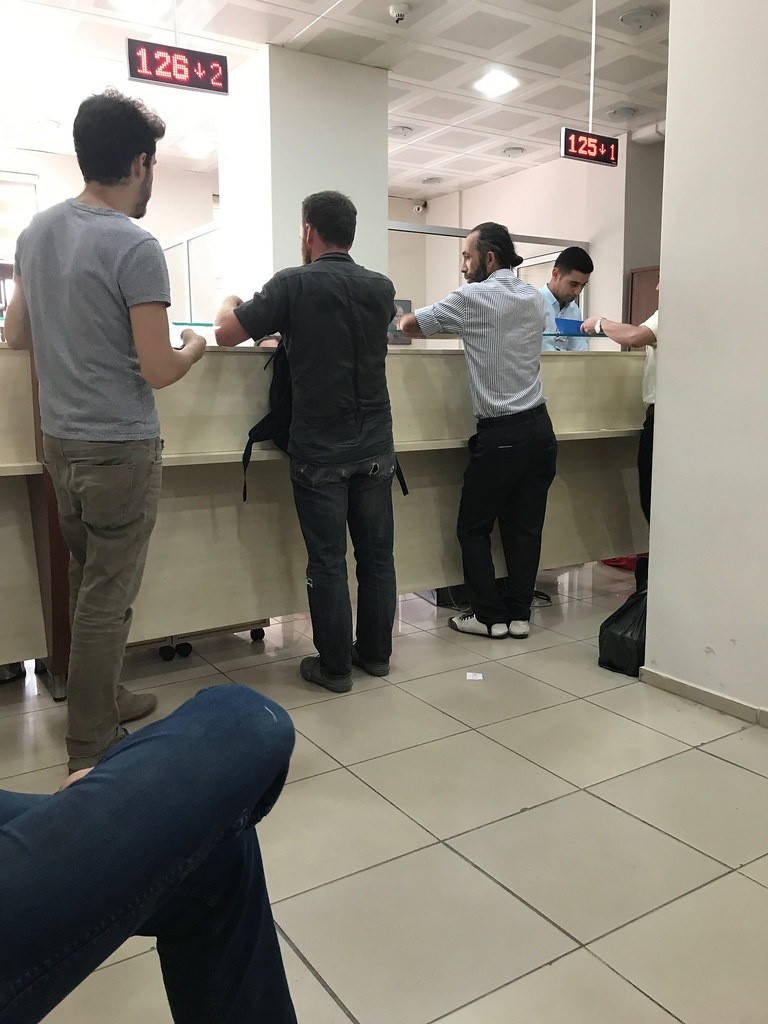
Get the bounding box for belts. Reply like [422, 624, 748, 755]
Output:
[478, 403, 547, 426]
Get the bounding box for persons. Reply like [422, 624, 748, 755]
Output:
[5, 91, 205, 775]
[579, 277, 660, 527]
[215, 190, 410, 693]
[0, 683, 306, 1023]
[535, 248, 595, 352]
[399, 221, 560, 639]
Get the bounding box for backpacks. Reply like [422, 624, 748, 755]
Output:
[247, 337, 293, 455]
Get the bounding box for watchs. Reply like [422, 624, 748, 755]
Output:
[595, 317, 606, 335]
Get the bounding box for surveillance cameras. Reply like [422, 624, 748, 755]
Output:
[413, 205, 422, 212]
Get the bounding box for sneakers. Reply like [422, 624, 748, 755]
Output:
[68, 732, 131, 774]
[509, 620, 529, 638]
[449, 611, 508, 638]
[300, 655, 352, 692]
[352, 640, 390, 676]
[116, 687, 156, 722]
